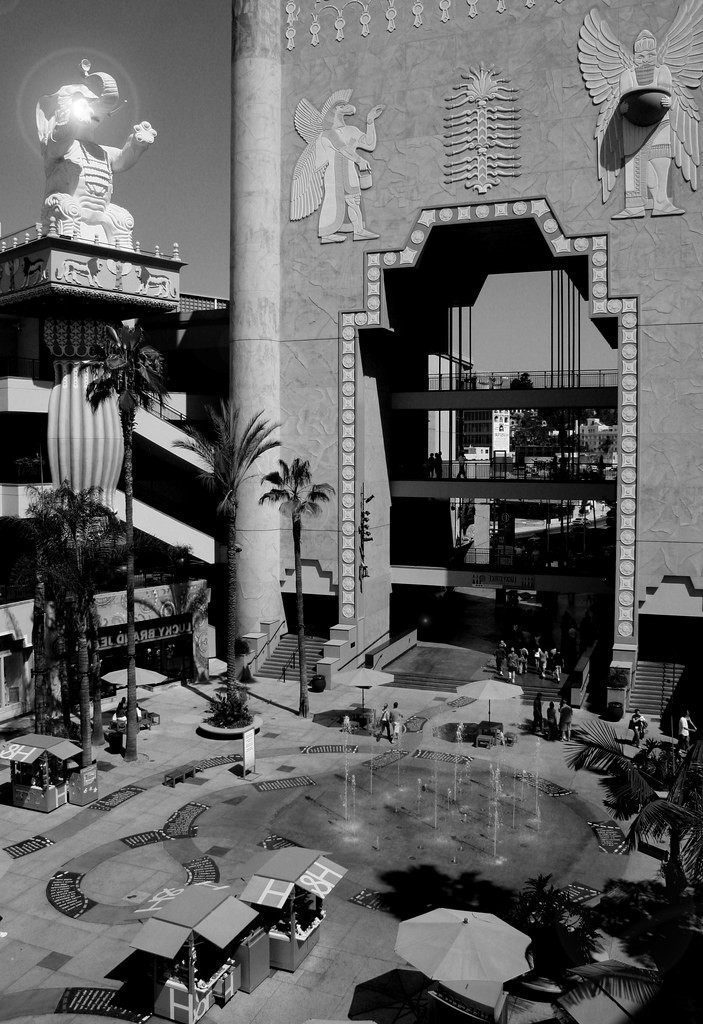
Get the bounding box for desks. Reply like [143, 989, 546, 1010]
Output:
[353, 707, 374, 718]
[477, 721, 503, 747]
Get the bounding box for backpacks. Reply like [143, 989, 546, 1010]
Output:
[534, 652, 540, 658]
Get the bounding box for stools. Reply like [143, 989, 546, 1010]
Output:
[147, 712, 160, 725]
[350, 720, 359, 733]
[463, 722, 517, 749]
[141, 708, 146, 718]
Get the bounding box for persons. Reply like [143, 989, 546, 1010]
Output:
[390, 702, 403, 744]
[533, 693, 573, 742]
[493, 623, 580, 683]
[559, 453, 569, 469]
[678, 709, 697, 743]
[428, 451, 468, 479]
[116, 697, 142, 724]
[632, 709, 646, 749]
[376, 703, 392, 742]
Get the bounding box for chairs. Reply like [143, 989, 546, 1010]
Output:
[109, 707, 151, 734]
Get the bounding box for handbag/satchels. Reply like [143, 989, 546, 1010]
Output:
[628, 720, 633, 729]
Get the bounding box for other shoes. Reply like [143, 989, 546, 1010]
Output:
[542, 673, 546, 678]
[636, 745, 641, 748]
[557, 677, 560, 682]
[512, 678, 515, 684]
[631, 742, 634, 746]
[376, 733, 381, 742]
[500, 671, 503, 676]
[391, 741, 395, 744]
[506, 679, 510, 682]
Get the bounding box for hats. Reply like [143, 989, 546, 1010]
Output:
[634, 709, 640, 713]
[511, 647, 514, 652]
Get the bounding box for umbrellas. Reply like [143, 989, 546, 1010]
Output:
[100, 667, 168, 685]
[331, 668, 394, 708]
[456, 679, 524, 722]
